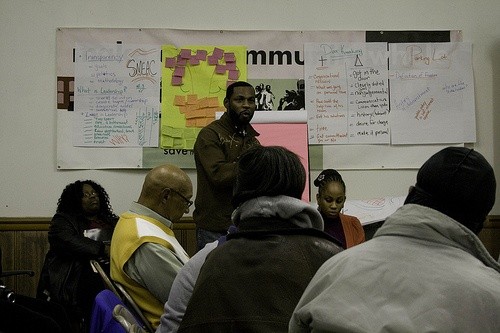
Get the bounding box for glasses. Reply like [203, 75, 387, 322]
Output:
[161, 187, 193, 209]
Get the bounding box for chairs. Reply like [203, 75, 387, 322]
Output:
[90, 260, 156, 333]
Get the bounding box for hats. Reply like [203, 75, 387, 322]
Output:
[414, 147, 496, 219]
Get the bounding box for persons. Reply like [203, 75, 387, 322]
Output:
[155, 146, 344, 333]
[192, 82, 261, 251]
[314, 168, 365, 250]
[289, 147, 500, 333]
[255, 79, 306, 111]
[110, 164, 192, 331]
[37, 180, 120, 333]
